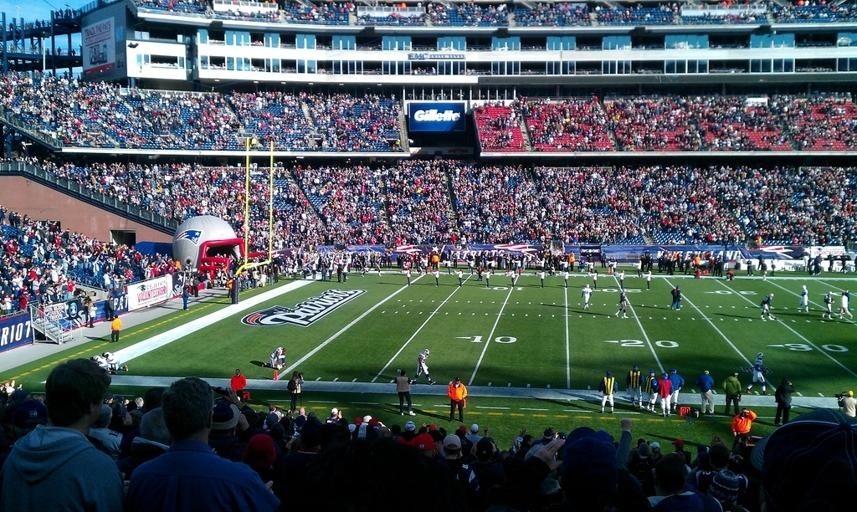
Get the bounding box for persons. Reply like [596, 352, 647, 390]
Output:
[0, 341, 857, 508]
[797, 284, 808, 313]
[0, 2, 247, 342]
[247, 2, 854, 292]
[613, 291, 628, 319]
[759, 293, 775, 321]
[579, 283, 592, 311]
[821, 289, 853, 320]
[669, 285, 681, 310]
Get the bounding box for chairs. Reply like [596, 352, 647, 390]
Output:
[14, 1, 854, 251]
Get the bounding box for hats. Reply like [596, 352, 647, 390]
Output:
[210, 402, 240, 429]
[752, 409, 855, 510]
[330, 408, 380, 434]
[405, 421, 478, 450]
[251, 435, 276, 466]
